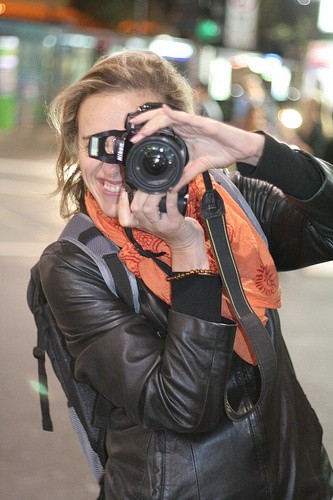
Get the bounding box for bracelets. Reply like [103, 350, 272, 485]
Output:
[164, 269, 215, 280]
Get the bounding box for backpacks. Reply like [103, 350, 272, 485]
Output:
[29, 212, 139, 486]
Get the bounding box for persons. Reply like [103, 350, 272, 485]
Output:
[23, 46, 332, 500]
[191, 70, 333, 164]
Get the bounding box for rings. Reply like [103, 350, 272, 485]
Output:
[149, 212, 164, 223]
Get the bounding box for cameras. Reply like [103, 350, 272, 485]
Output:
[87, 103, 190, 217]
